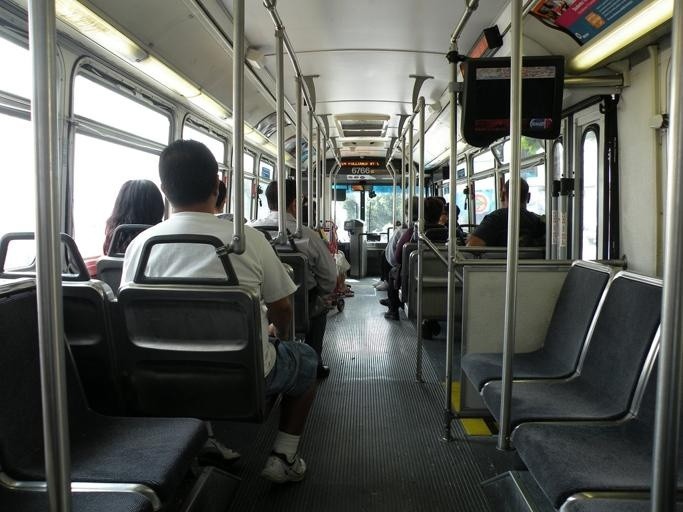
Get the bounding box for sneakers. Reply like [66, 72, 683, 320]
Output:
[385, 312, 399, 321]
[380, 299, 389, 306]
[260, 450, 306, 483]
[372, 280, 389, 291]
[204, 438, 241, 459]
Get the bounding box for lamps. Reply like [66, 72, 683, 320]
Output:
[565, 0, 674, 73]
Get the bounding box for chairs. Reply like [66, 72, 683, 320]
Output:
[394, 243, 550, 343]
[393, 242, 683, 512]
[42, 223, 317, 469]
[1, 280, 242, 511]
[457, 255, 681, 512]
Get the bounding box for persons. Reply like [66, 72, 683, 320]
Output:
[117, 139, 318, 485]
[243, 179, 335, 384]
[100, 179, 163, 258]
[213, 180, 226, 214]
[372, 196, 462, 340]
[297, 198, 354, 297]
[465, 177, 545, 248]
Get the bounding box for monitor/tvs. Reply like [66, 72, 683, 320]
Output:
[460, 55, 564, 146]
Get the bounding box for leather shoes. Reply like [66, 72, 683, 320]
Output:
[323, 365, 330, 373]
